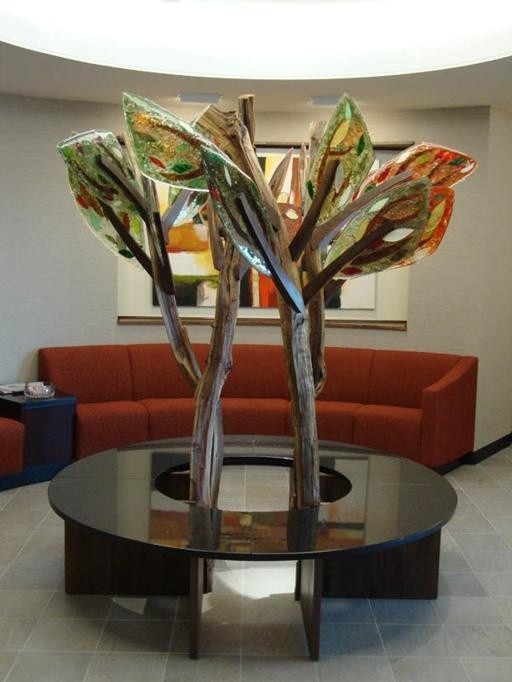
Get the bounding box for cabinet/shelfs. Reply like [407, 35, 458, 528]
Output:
[0, 393, 77, 491]
